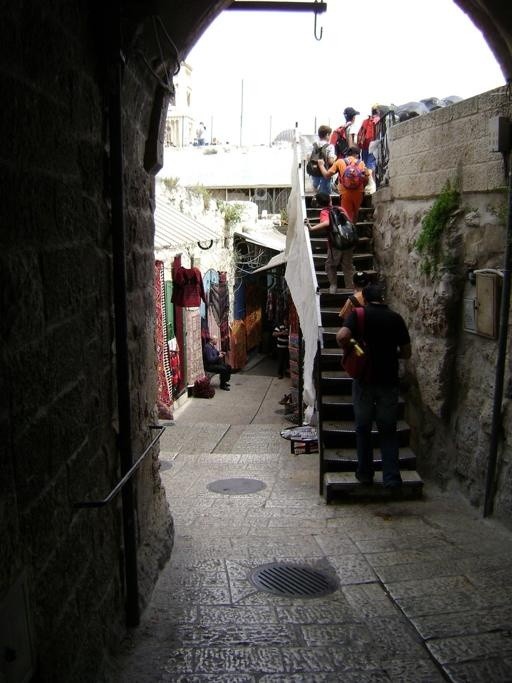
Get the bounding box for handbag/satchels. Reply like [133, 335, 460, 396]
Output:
[339, 341, 365, 379]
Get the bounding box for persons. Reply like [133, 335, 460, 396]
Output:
[338, 271, 373, 319]
[194, 120, 219, 145]
[203, 334, 234, 392]
[307, 104, 391, 224]
[301, 191, 356, 294]
[272, 315, 290, 380]
[333, 279, 413, 493]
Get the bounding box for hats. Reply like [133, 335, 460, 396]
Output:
[347, 144, 360, 154]
[353, 271, 368, 282]
[344, 107, 360, 117]
[316, 191, 330, 204]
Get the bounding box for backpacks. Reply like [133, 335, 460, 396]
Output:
[307, 142, 330, 176]
[327, 205, 358, 249]
[330, 123, 352, 144]
[342, 157, 365, 189]
